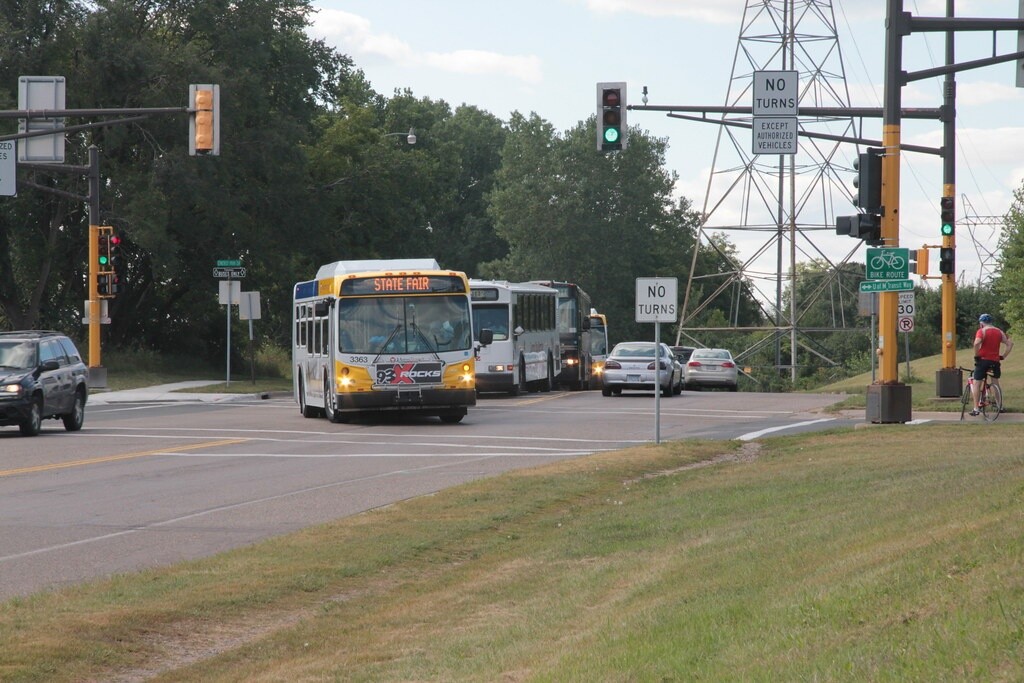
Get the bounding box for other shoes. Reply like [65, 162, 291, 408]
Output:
[968, 410, 980, 416]
[997, 408, 1005, 413]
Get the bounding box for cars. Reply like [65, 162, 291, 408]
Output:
[685, 348, 741, 392]
[668, 346, 697, 390]
[600, 341, 684, 398]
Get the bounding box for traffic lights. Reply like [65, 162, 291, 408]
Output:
[835, 213, 862, 241]
[596, 83, 627, 151]
[940, 197, 954, 236]
[111, 273, 120, 294]
[908, 249, 928, 275]
[939, 248, 956, 276]
[97, 235, 109, 267]
[109, 235, 121, 266]
[853, 153, 881, 210]
[96, 273, 108, 295]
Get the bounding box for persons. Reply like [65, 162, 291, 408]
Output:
[969, 314, 1014, 415]
[427, 310, 454, 339]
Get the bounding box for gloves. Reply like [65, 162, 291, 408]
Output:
[999, 356, 1004, 361]
[973, 355, 981, 360]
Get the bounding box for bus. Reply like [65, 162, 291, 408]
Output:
[291, 258, 493, 424]
[590, 308, 609, 390]
[468, 277, 562, 397]
[528, 280, 592, 392]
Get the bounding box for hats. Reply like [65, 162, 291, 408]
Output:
[978, 313, 994, 322]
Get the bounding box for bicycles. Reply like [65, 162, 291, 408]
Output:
[957, 365, 1002, 423]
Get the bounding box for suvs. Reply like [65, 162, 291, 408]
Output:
[0, 330, 89, 437]
